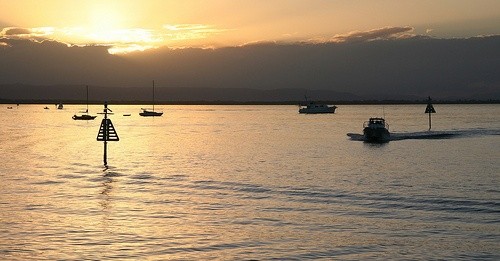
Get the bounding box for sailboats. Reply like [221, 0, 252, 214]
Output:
[139, 78, 163, 116]
[72, 84, 97, 120]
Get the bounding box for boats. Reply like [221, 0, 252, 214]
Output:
[298, 94, 339, 115]
[361, 117, 391, 143]
